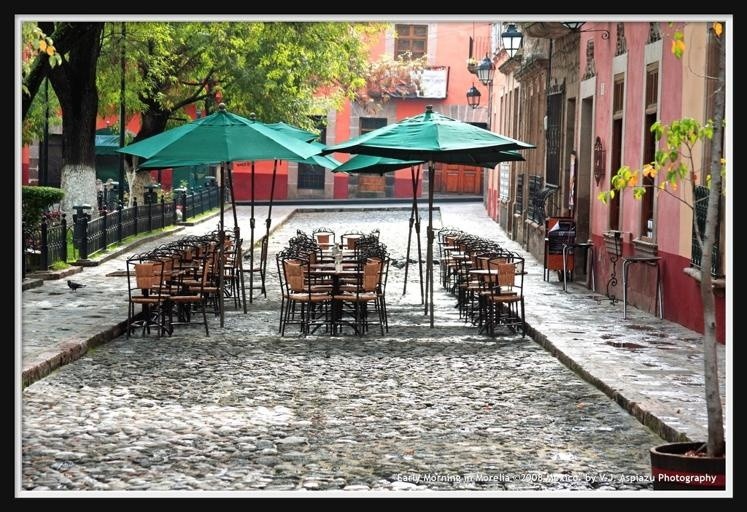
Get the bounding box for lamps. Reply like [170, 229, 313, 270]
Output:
[475, 51, 503, 86]
[467, 82, 491, 108]
[562, 22, 611, 40]
[501, 25, 525, 60]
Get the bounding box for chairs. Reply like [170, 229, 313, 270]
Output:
[124, 229, 268, 336]
[276, 227, 391, 338]
[436, 226, 528, 339]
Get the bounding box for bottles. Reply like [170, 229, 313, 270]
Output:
[333, 243, 340, 254]
[334, 254, 344, 272]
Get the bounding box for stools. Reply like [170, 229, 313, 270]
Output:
[561, 242, 595, 293]
[621, 255, 666, 322]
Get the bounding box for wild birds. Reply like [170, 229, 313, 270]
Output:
[391, 258, 418, 270]
[244, 255, 251, 260]
[68, 280, 85, 293]
[421, 259, 425, 263]
[433, 260, 439, 264]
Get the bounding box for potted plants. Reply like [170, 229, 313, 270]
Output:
[596, 22, 726, 490]
